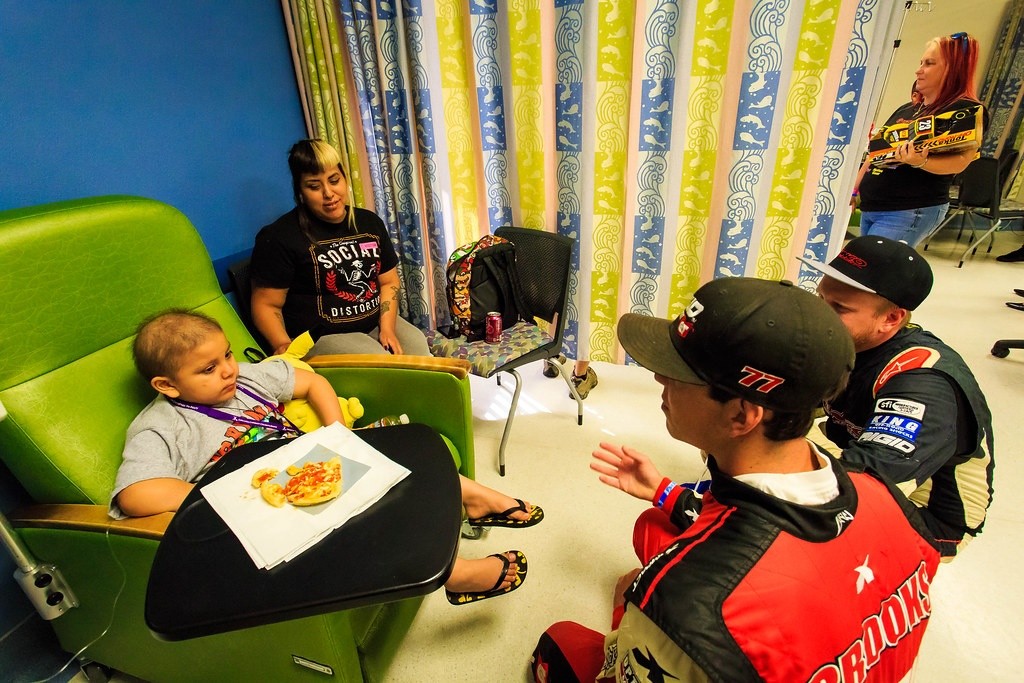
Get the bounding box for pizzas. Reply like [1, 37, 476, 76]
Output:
[252, 454, 342, 507]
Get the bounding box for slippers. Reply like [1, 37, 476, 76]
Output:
[445, 550, 528, 605]
[468, 498, 543, 528]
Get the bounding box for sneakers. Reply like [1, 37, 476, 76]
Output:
[569, 367, 599, 400]
[543, 354, 566, 377]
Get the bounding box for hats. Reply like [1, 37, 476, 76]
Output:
[797, 236, 933, 310]
[617, 278, 853, 417]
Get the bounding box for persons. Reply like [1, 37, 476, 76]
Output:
[849, 32, 990, 250]
[530, 277, 941, 683]
[107, 307, 544, 606]
[796, 236, 995, 564]
[250, 138, 430, 355]
[542, 355, 598, 399]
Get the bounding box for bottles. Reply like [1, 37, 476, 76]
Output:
[359, 414, 409, 429]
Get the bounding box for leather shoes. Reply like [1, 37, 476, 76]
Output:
[1006, 303, 1024, 311]
[1014, 289, 1024, 297]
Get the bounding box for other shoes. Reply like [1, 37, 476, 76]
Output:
[996, 247, 1024, 262]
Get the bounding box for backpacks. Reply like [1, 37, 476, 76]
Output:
[444, 234, 521, 343]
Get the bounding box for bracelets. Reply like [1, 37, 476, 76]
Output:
[912, 158, 928, 168]
[852, 194, 857, 196]
[657, 481, 677, 509]
[852, 190, 858, 194]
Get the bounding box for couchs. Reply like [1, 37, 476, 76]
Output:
[0, 193, 476, 683]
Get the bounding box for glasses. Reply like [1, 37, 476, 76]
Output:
[950, 32, 968, 60]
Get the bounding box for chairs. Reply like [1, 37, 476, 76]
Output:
[226, 258, 270, 348]
[924, 148, 1024, 268]
[425, 226, 584, 477]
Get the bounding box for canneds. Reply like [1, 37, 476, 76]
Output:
[485, 311, 502, 344]
